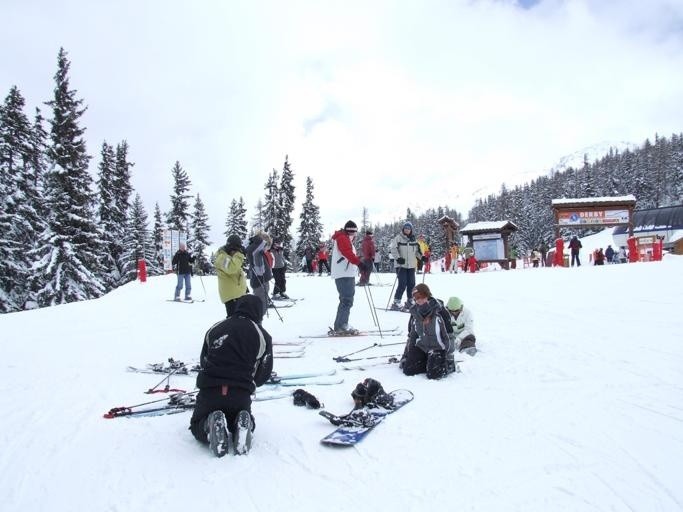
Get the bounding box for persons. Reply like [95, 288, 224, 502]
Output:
[189, 294, 274, 461]
[330, 220, 369, 336]
[389, 221, 429, 313]
[301, 233, 480, 277]
[510, 233, 631, 269]
[243, 229, 273, 319]
[444, 295, 477, 356]
[355, 229, 376, 286]
[214, 233, 247, 320]
[269, 243, 292, 300]
[168, 243, 195, 302]
[397, 283, 457, 380]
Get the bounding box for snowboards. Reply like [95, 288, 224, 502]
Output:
[322, 389, 414, 445]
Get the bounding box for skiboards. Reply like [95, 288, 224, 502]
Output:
[128, 358, 344, 385]
[375, 307, 410, 313]
[167, 298, 205, 303]
[300, 326, 404, 338]
[333, 354, 404, 370]
[267, 304, 294, 308]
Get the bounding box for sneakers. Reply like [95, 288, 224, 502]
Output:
[174, 296, 180, 303]
[392, 300, 408, 313]
[280, 291, 289, 299]
[358, 279, 371, 286]
[334, 325, 360, 335]
[403, 299, 416, 310]
[272, 291, 283, 300]
[232, 410, 253, 455]
[185, 295, 192, 299]
[206, 411, 227, 457]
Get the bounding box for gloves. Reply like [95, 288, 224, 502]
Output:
[237, 245, 247, 256]
[396, 257, 406, 264]
[423, 255, 429, 262]
[358, 262, 369, 273]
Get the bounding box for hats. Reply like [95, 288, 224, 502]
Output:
[226, 235, 243, 248]
[402, 222, 413, 230]
[366, 230, 373, 234]
[447, 296, 461, 311]
[179, 243, 185, 250]
[345, 220, 358, 232]
